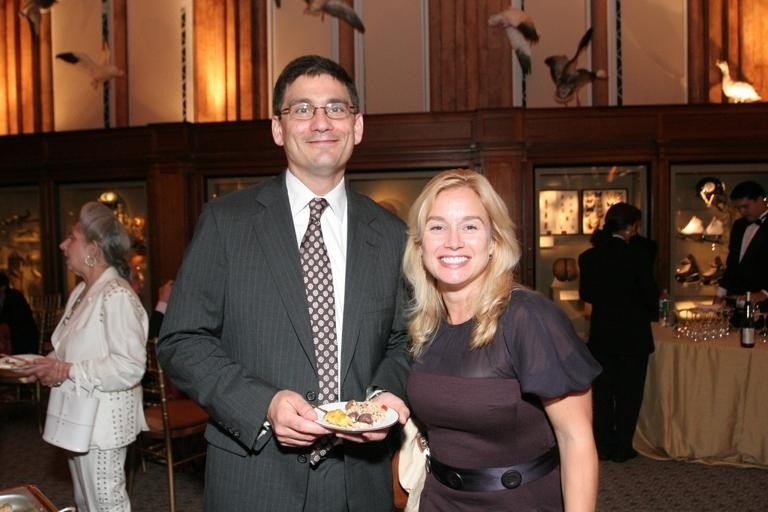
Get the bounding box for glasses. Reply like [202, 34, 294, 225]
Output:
[280, 102, 357, 119]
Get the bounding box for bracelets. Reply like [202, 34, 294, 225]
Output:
[365, 386, 390, 404]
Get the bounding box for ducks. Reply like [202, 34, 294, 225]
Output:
[712, 57, 761, 102]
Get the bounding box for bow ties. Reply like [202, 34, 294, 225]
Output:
[747, 219, 762, 226]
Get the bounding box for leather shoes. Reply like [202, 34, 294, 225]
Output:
[599, 448, 637, 462]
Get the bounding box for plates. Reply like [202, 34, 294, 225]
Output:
[306, 400, 400, 434]
[0, 352, 47, 375]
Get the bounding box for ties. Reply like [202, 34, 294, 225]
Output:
[298, 198, 338, 465]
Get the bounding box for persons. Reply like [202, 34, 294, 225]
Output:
[405, 169, 604, 511]
[578, 204, 660, 464]
[146, 278, 173, 408]
[714, 180, 767, 333]
[154, 53, 422, 510]
[0, 271, 41, 355]
[634, 209, 659, 273]
[13, 201, 155, 510]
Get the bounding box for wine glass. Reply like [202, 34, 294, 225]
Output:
[671, 305, 733, 342]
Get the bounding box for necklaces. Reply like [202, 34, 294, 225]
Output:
[72, 282, 90, 311]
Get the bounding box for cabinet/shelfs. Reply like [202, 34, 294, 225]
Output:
[536, 169, 649, 350]
[201, 163, 471, 232]
[668, 161, 768, 309]
[1, 174, 158, 310]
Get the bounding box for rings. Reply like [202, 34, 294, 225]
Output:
[55, 381, 61, 387]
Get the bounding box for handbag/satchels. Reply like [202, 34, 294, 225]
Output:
[42, 387, 100, 454]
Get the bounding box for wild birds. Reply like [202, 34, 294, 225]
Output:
[486, 8, 542, 74]
[295, 0, 366, 35]
[54, 42, 127, 91]
[544, 27, 609, 107]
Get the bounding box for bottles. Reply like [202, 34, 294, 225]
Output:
[741, 292, 756, 348]
[658, 289, 673, 329]
